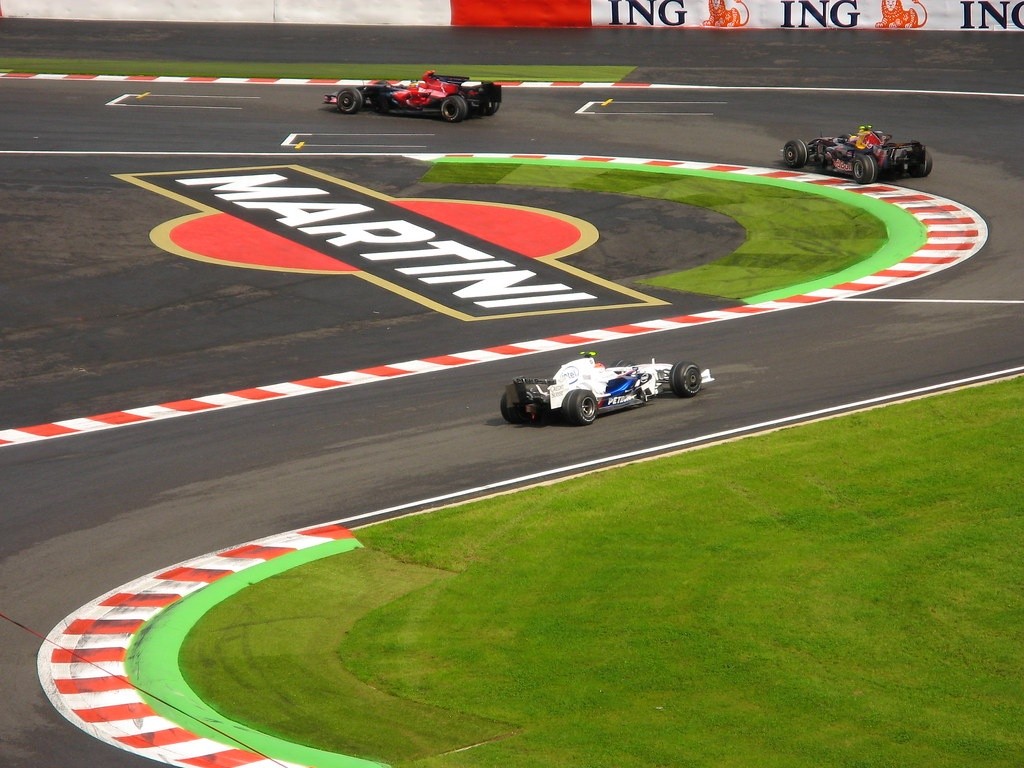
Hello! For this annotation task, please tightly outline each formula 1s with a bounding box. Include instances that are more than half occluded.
[784,124,935,186]
[322,66,504,121]
[501,348,715,427]
